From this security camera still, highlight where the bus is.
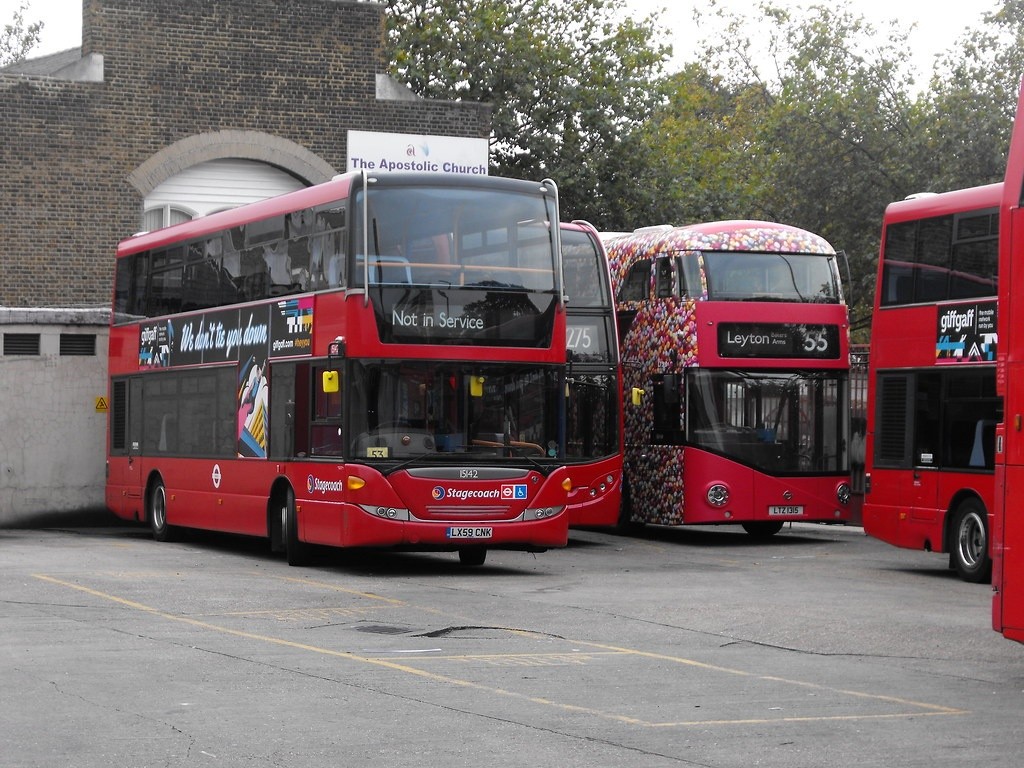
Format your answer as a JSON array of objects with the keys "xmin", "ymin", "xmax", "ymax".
[
  {"xmin": 601, "ymin": 217, "xmax": 855, "ymax": 541},
  {"xmin": 393, "ymin": 217, "xmax": 625, "ymax": 530},
  {"xmin": 990, "ymin": 66, "xmax": 1024, "ymax": 645},
  {"xmin": 103, "ymin": 164, "xmax": 567, "ymax": 565},
  {"xmin": 862, "ymin": 183, "xmax": 1006, "ymax": 591}
]
